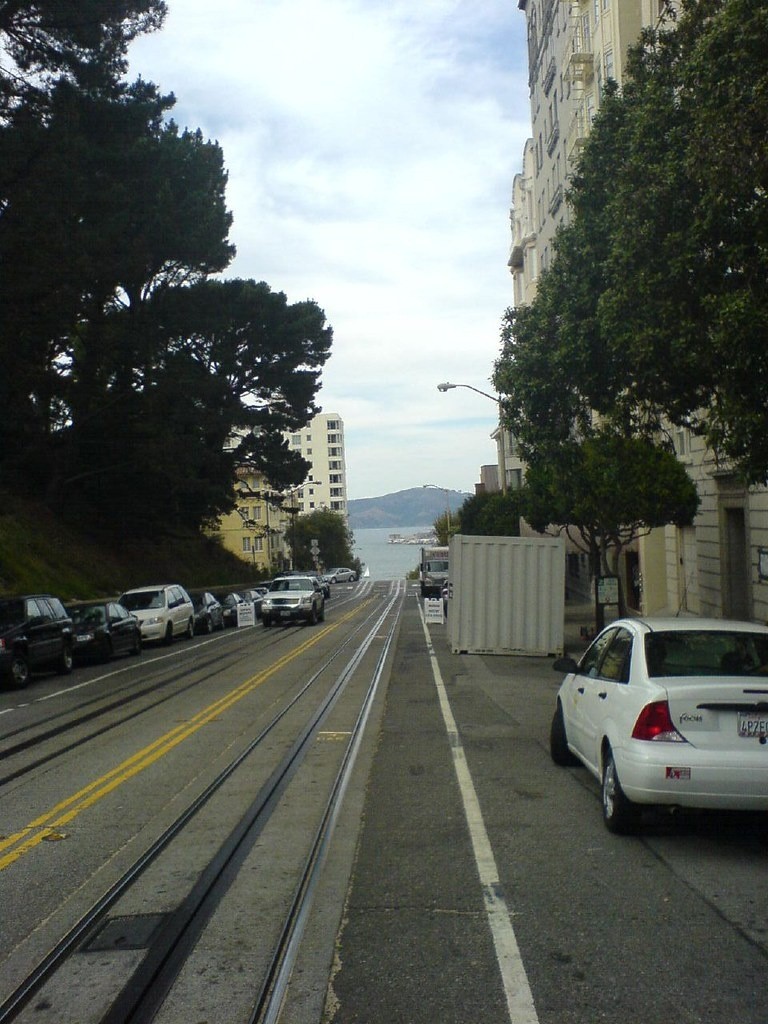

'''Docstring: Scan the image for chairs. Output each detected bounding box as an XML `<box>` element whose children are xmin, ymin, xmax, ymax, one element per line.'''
<box><xmin>721</xmin><ymin>649</ymin><xmax>758</xmax><ymax>676</ymax></box>
<box><xmin>616</xmin><ymin>645</ymin><xmax>669</xmax><ymax>684</ymax></box>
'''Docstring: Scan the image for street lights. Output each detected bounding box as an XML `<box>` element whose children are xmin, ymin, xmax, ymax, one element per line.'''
<box><xmin>423</xmin><ymin>483</ymin><xmax>451</xmax><ymax>542</ymax></box>
<box><xmin>434</xmin><ymin>381</ymin><xmax>509</xmax><ymax>497</ymax></box>
<box><xmin>291</xmin><ymin>481</ymin><xmax>324</xmax><ymax>567</ymax></box>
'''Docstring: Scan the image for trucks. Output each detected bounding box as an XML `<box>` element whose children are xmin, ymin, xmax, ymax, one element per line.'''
<box><xmin>419</xmin><ymin>545</ymin><xmax>449</xmax><ymax>598</ymax></box>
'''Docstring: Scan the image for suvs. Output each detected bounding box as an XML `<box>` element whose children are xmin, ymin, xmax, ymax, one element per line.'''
<box><xmin>116</xmin><ymin>584</ymin><xmax>195</xmax><ymax>646</ymax></box>
<box><xmin>0</xmin><ymin>594</ymin><xmax>77</xmax><ymax>689</ymax></box>
<box><xmin>259</xmin><ymin>576</ymin><xmax>325</xmax><ymax>624</ymax></box>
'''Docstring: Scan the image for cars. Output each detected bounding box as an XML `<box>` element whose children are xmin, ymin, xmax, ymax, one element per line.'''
<box><xmin>186</xmin><ymin>591</ymin><xmax>227</xmax><ymax>634</ymax></box>
<box><xmin>322</xmin><ymin>568</ymin><xmax>358</xmax><ymax>584</ymax></box>
<box><xmin>277</xmin><ymin>569</ymin><xmax>331</xmax><ymax>598</ymax></box>
<box><xmin>235</xmin><ymin>581</ymin><xmax>272</xmax><ymax>618</ymax></box>
<box><xmin>215</xmin><ymin>592</ymin><xmax>246</xmax><ymax>626</ymax></box>
<box><xmin>550</xmin><ymin>616</ymin><xmax>768</xmax><ymax>835</ymax></box>
<box><xmin>67</xmin><ymin>602</ymin><xmax>143</xmax><ymax>668</ymax></box>
<box><xmin>439</xmin><ymin>577</ymin><xmax>448</xmax><ymax>609</ymax></box>
